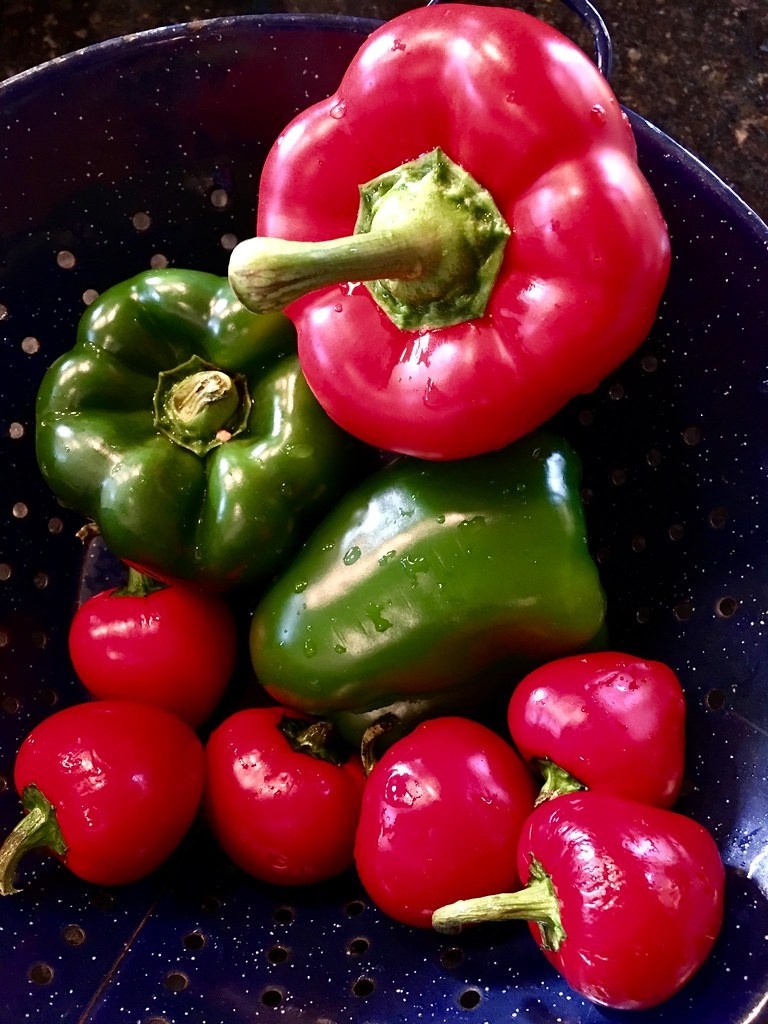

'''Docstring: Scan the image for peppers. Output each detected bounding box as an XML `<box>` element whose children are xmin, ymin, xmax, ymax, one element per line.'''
<box><xmin>1</xmin><ymin>7</ymin><xmax>727</xmax><ymax>1011</ymax></box>
